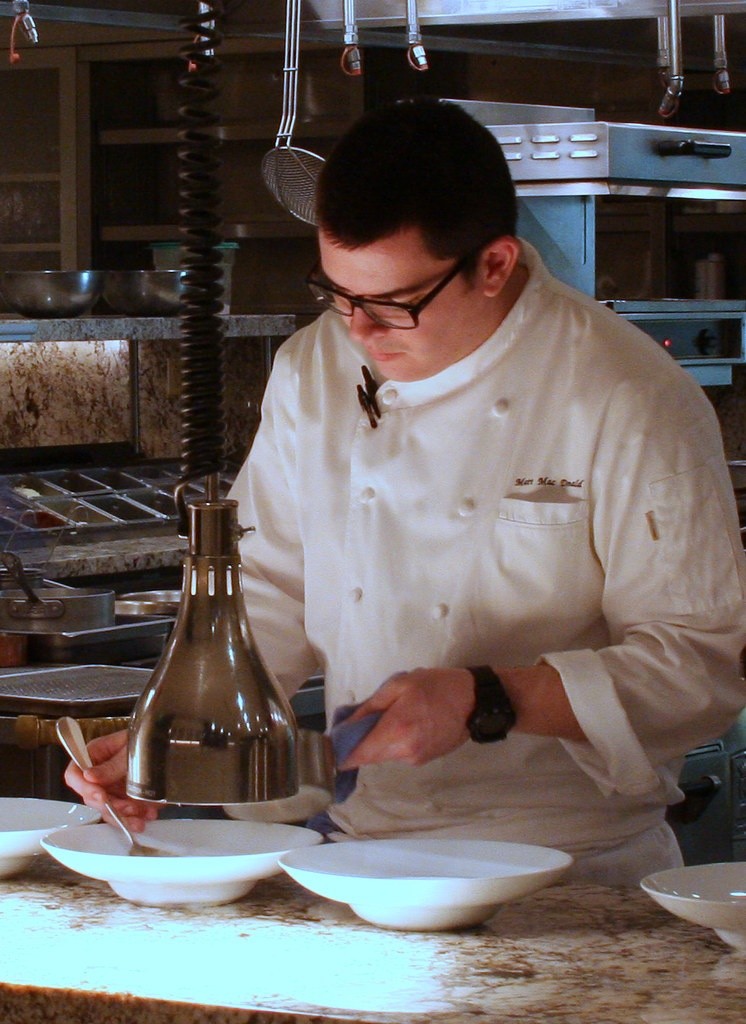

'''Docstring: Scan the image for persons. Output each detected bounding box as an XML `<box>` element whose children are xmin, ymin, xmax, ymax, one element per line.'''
<box><xmin>64</xmin><ymin>94</ymin><xmax>746</xmax><ymax>889</ymax></box>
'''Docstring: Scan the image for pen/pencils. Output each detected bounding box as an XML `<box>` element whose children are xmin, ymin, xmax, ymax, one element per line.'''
<box><xmin>357</xmin><ymin>384</ymin><xmax>377</xmax><ymax>429</ymax></box>
<box><xmin>361</xmin><ymin>365</ymin><xmax>382</xmax><ymax>419</ymax></box>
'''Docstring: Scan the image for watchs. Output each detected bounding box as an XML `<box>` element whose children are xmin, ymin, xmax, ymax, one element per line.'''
<box><xmin>465</xmin><ymin>665</ymin><xmax>516</xmax><ymax>744</ymax></box>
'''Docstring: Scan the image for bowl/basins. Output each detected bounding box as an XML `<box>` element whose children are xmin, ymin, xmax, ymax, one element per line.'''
<box><xmin>0</xmin><ymin>269</ymin><xmax>107</xmax><ymax>319</ymax></box>
<box><xmin>102</xmin><ymin>269</ymin><xmax>189</xmax><ymax>316</ymax></box>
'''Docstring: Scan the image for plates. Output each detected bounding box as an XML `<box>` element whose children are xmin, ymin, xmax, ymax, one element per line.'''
<box><xmin>40</xmin><ymin>818</ymin><xmax>322</xmax><ymax>908</ymax></box>
<box><xmin>0</xmin><ymin>797</ymin><xmax>102</xmax><ymax>879</ymax></box>
<box><xmin>277</xmin><ymin>838</ymin><xmax>575</xmax><ymax>931</ymax></box>
<box><xmin>641</xmin><ymin>859</ymin><xmax>746</xmax><ymax>948</ymax></box>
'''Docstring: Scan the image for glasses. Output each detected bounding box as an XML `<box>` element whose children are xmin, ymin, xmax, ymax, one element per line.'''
<box><xmin>304</xmin><ymin>254</ymin><xmax>467</xmax><ymax>330</ymax></box>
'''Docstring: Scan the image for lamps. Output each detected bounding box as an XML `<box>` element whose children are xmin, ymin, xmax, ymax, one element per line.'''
<box><xmin>125</xmin><ymin>0</ymin><xmax>305</xmax><ymax>806</ymax></box>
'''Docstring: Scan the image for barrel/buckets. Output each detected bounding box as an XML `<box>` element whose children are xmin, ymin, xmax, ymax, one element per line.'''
<box><xmin>0</xmin><ymin>586</ymin><xmax>117</xmax><ymax>632</ymax></box>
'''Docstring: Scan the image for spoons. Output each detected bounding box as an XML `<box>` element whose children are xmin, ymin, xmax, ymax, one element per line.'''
<box><xmin>55</xmin><ymin>716</ymin><xmax>177</xmax><ymax>857</ymax></box>
